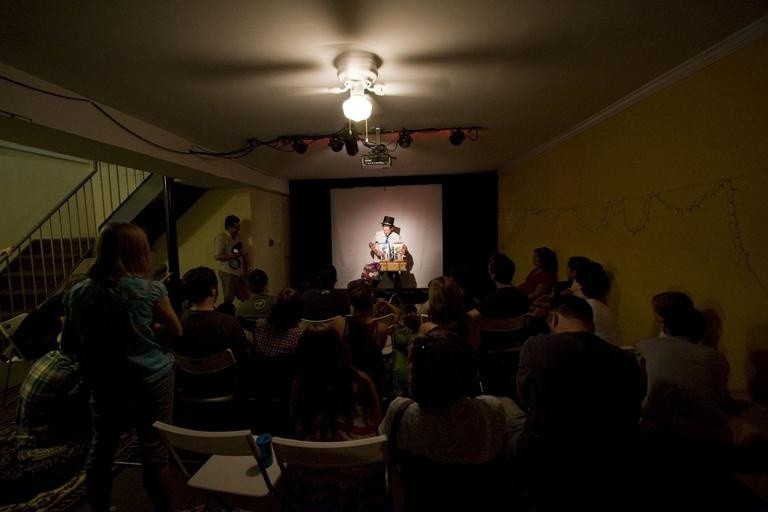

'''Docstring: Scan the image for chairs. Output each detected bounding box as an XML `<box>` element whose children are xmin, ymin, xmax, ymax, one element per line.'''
<box><xmin>270</xmin><ymin>433</ymin><xmax>390</xmax><ymax>512</ymax></box>
<box><xmin>154</xmin><ymin>423</ymin><xmax>287</xmax><ymax>511</ymax></box>
<box><xmin>0</xmin><ymin>309</ymin><xmax>526</xmax><ymax>411</ymax></box>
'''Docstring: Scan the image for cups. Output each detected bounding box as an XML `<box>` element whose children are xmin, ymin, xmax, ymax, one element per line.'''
<box><xmin>256</xmin><ymin>434</ymin><xmax>272</xmax><ymax>467</ymax></box>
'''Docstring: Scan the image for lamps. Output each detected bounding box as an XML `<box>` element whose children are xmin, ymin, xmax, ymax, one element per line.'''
<box><xmin>340</xmin><ymin>88</ymin><xmax>373</xmax><ymax>144</ymax></box>
<box><xmin>277</xmin><ymin>124</ymin><xmax>472</xmax><ymax>156</ymax></box>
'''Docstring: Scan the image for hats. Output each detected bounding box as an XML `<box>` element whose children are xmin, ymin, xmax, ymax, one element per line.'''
<box><xmin>381</xmin><ymin>216</ymin><xmax>395</xmax><ymax>227</ymax></box>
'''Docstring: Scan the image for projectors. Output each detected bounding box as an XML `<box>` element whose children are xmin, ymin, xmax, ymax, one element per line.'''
<box><xmin>361</xmin><ymin>153</ymin><xmax>394</xmax><ymax>170</ymax></box>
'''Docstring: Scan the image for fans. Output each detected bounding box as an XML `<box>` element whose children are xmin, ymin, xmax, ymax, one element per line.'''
<box><xmin>264</xmin><ymin>47</ymin><xmax>451</xmax><ymax>99</ymax></box>
